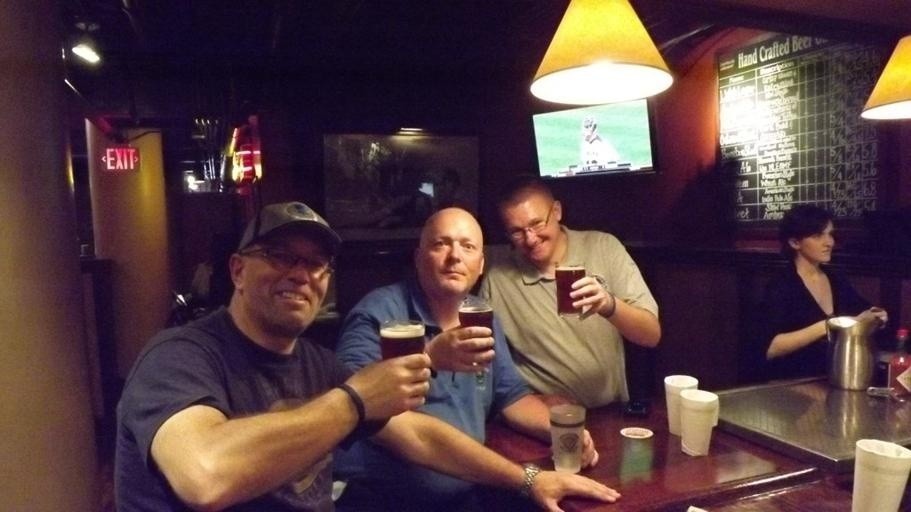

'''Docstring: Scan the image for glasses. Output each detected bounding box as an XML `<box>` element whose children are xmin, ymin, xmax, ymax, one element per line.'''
<box><xmin>242</xmin><ymin>248</ymin><xmax>334</xmax><ymax>276</ymax></box>
<box><xmin>507</xmin><ymin>200</ymin><xmax>555</xmax><ymax>241</ymax></box>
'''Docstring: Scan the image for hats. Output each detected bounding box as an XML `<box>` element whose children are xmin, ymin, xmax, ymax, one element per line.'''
<box><xmin>238</xmin><ymin>202</ymin><xmax>343</xmax><ymax>264</ymax></box>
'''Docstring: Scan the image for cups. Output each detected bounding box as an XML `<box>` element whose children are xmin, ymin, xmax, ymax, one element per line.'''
<box><xmin>680</xmin><ymin>390</ymin><xmax>718</xmax><ymax>456</ymax></box>
<box><xmin>378</xmin><ymin>318</ymin><xmax>425</xmax><ymax>365</ymax></box>
<box><xmin>852</xmin><ymin>439</ymin><xmax>911</xmax><ymax>512</ymax></box>
<box><xmin>555</xmin><ymin>262</ymin><xmax>585</xmax><ymax>317</ymax></box>
<box><xmin>665</xmin><ymin>376</ymin><xmax>698</xmax><ymax>436</ymax></box>
<box><xmin>459</xmin><ymin>298</ymin><xmax>495</xmax><ymax>363</ymax></box>
<box><xmin>551</xmin><ymin>402</ymin><xmax>586</xmax><ymax>474</ymax></box>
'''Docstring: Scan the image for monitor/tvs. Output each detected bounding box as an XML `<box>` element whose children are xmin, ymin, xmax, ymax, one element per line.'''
<box><xmin>532</xmin><ymin>96</ymin><xmax>657</xmax><ymax>189</ymax></box>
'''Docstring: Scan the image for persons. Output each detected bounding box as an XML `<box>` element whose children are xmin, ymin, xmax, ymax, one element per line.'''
<box><xmin>186</xmin><ymin>232</ymin><xmax>219</xmax><ymax>300</ymax></box>
<box><xmin>325</xmin><ymin>138</ymin><xmax>412</xmax><ymax>230</ymax></box>
<box><xmin>748</xmin><ymin>205</ymin><xmax>888</xmax><ymax>378</ymax></box>
<box><xmin>574</xmin><ymin>114</ymin><xmax>626</xmax><ymax>169</ymax></box>
<box><xmin>477</xmin><ymin>180</ymin><xmax>662</xmax><ymax>409</ymax></box>
<box><xmin>331</xmin><ymin>207</ymin><xmax>602</xmax><ymax>512</ymax></box>
<box><xmin>113</xmin><ymin>203</ymin><xmax>619</xmax><ymax>512</ymax></box>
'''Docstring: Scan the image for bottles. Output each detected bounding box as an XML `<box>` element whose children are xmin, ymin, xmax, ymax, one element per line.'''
<box><xmin>887</xmin><ymin>327</ymin><xmax>911</xmax><ymax>398</ymax></box>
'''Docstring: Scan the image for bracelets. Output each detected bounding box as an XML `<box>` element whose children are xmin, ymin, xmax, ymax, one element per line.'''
<box><xmin>520</xmin><ymin>462</ymin><xmax>540</xmax><ymax>500</ymax></box>
<box><xmin>422</xmin><ymin>353</ymin><xmax>438</xmax><ymax>381</ymax></box>
<box><xmin>599</xmin><ymin>292</ymin><xmax>615</xmax><ymax>319</ymax></box>
<box><xmin>337</xmin><ymin>383</ymin><xmax>365</xmax><ymax>427</ymax></box>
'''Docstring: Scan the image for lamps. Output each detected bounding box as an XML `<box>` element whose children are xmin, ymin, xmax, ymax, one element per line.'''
<box><xmin>858</xmin><ymin>36</ymin><xmax>911</xmax><ymax>120</ymax></box>
<box><xmin>529</xmin><ymin>0</ymin><xmax>675</xmax><ymax>106</ymax></box>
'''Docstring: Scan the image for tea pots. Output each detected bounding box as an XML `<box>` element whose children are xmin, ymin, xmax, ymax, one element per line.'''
<box><xmin>826</xmin><ymin>314</ymin><xmax>882</xmax><ymax>391</ymax></box>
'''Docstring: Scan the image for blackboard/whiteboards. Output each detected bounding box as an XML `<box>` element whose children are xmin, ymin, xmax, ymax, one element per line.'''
<box><xmin>714</xmin><ymin>32</ymin><xmax>899</xmax><ymax>227</ymax></box>
